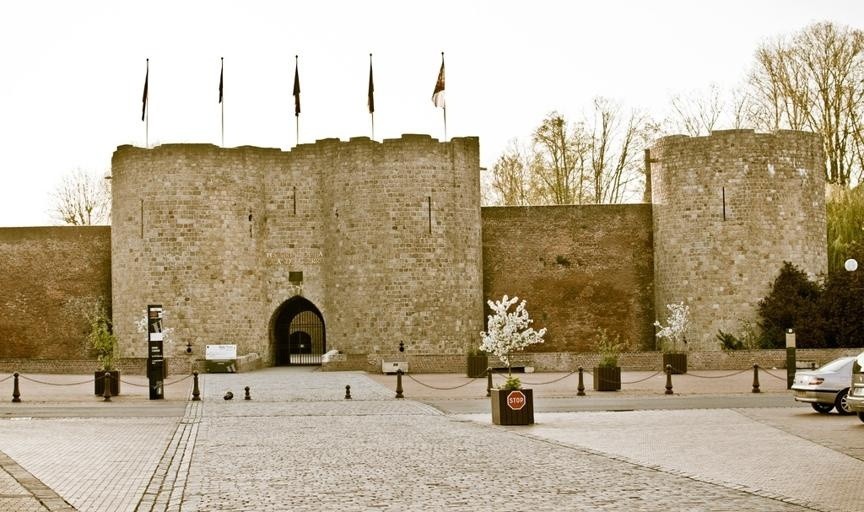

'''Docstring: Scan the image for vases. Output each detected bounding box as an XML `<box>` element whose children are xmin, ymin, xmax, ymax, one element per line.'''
<box><xmin>490</xmin><ymin>388</ymin><xmax>534</xmax><ymax>425</ymax></box>
<box><xmin>663</xmin><ymin>354</ymin><xmax>687</xmax><ymax>374</ymax></box>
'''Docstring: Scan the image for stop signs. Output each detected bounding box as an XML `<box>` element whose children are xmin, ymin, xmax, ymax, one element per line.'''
<box><xmin>507</xmin><ymin>391</ymin><xmax>527</xmax><ymax>411</ymax></box>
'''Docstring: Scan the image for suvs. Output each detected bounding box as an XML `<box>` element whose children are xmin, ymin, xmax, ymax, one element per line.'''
<box><xmin>847</xmin><ymin>353</ymin><xmax>864</xmax><ymax>422</ymax></box>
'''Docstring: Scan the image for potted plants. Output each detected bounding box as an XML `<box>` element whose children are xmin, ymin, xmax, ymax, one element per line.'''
<box><xmin>87</xmin><ymin>311</ymin><xmax>120</xmax><ymax>395</ymax></box>
<box><xmin>593</xmin><ymin>329</ymin><xmax>627</xmax><ymax>390</ymax></box>
<box><xmin>464</xmin><ymin>333</ymin><xmax>489</xmax><ymax>379</ymax></box>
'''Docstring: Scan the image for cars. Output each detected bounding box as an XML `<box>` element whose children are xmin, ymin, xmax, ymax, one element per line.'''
<box><xmin>792</xmin><ymin>356</ymin><xmax>859</xmax><ymax>414</ymax></box>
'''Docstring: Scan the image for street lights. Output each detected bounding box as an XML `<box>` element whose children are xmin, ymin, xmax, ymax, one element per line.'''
<box><xmin>842</xmin><ymin>259</ymin><xmax>860</xmax><ymax>284</ymax></box>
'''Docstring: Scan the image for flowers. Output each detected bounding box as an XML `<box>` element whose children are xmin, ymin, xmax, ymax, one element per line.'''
<box><xmin>479</xmin><ymin>295</ymin><xmax>547</xmax><ymax>388</ymax></box>
<box><xmin>653</xmin><ymin>301</ymin><xmax>692</xmax><ymax>354</ymax></box>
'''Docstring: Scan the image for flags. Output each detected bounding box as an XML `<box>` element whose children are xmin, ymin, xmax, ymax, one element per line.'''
<box><xmin>219</xmin><ymin>57</ymin><xmax>224</xmax><ymax>103</ymax></box>
<box><xmin>368</xmin><ymin>53</ymin><xmax>374</xmax><ymax>113</ymax></box>
<box><xmin>293</xmin><ymin>55</ymin><xmax>300</xmax><ymax>116</ymax></box>
<box><xmin>142</xmin><ymin>58</ymin><xmax>149</xmax><ymax>121</ymax></box>
<box><xmin>431</xmin><ymin>52</ymin><xmax>446</xmax><ymax>108</ymax></box>
